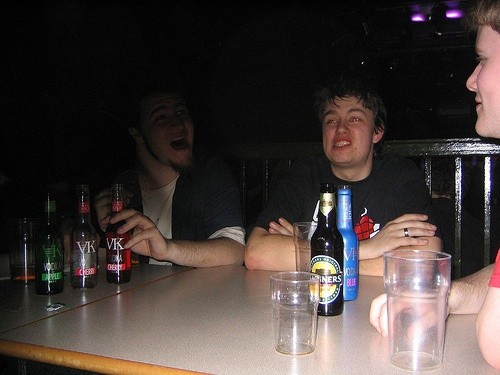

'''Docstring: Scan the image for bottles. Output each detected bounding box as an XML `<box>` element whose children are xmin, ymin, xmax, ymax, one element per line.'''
<box><xmin>307</xmin><ymin>183</ymin><xmax>344</xmax><ymax>317</ymax></box>
<box><xmin>70</xmin><ymin>184</ymin><xmax>98</xmax><ymax>289</ymax></box>
<box><xmin>332</xmin><ymin>184</ymin><xmax>359</xmax><ymax>300</ymax></box>
<box><xmin>34</xmin><ymin>189</ymin><xmax>65</xmax><ymax>295</ymax></box>
<box><xmin>104</xmin><ymin>182</ymin><xmax>132</xmax><ymax>284</ymax></box>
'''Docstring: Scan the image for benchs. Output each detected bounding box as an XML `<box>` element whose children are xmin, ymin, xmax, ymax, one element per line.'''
<box><xmin>199</xmin><ymin>136</ymin><xmax>500</xmax><ymax>280</ymax></box>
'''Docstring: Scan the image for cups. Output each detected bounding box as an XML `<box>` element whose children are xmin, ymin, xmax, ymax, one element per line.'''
<box><xmin>8</xmin><ymin>217</ymin><xmax>38</xmax><ymax>281</ymax></box>
<box><xmin>269</xmin><ymin>270</ymin><xmax>320</xmax><ymax>355</ymax></box>
<box><xmin>384</xmin><ymin>249</ymin><xmax>453</xmax><ymax>371</ymax></box>
<box><xmin>293</xmin><ymin>222</ymin><xmax>321</xmax><ymax>298</ymax></box>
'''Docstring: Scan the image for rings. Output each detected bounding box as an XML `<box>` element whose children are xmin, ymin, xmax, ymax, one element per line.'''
<box><xmin>404</xmin><ymin>228</ymin><xmax>409</xmax><ymax>237</ymax></box>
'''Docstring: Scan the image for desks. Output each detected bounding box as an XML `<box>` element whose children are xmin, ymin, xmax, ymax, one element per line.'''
<box><xmin>0</xmin><ymin>265</ymin><xmax>498</xmax><ymax>375</ymax></box>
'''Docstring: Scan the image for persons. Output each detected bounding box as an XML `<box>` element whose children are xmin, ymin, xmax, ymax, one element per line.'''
<box><xmin>244</xmin><ymin>72</ymin><xmax>442</xmax><ymax>277</ymax></box>
<box><xmin>93</xmin><ymin>80</ymin><xmax>246</xmax><ymax>267</ymax></box>
<box><xmin>368</xmin><ymin>0</ymin><xmax>500</xmax><ymax>370</ymax></box>
